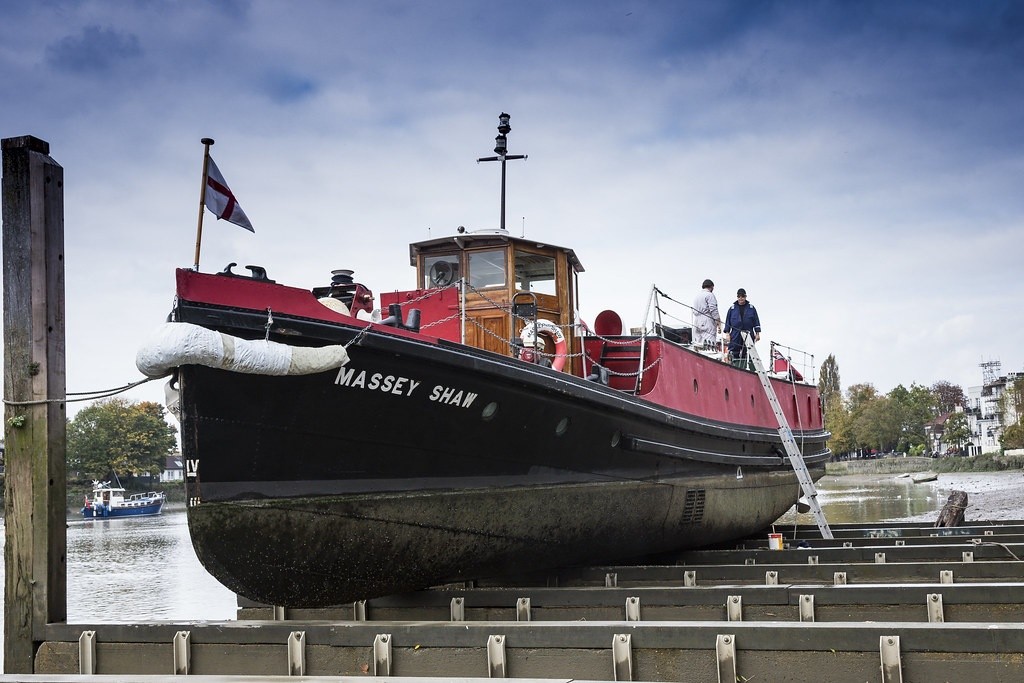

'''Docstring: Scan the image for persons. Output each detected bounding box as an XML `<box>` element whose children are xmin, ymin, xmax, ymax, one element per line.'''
<box><xmin>723</xmin><ymin>288</ymin><xmax>761</xmax><ymax>370</ymax></box>
<box><xmin>921</xmin><ymin>449</ymin><xmax>938</xmax><ymax>457</ymax></box>
<box><xmin>692</xmin><ymin>279</ymin><xmax>721</xmax><ymax>349</ymax></box>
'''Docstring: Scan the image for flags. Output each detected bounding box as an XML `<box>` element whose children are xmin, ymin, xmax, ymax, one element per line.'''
<box><xmin>774</xmin><ymin>351</ymin><xmax>804</xmax><ymax>381</ymax></box>
<box><xmin>203</xmin><ymin>155</ymin><xmax>255</xmax><ymax>232</ymax></box>
<box><xmin>85</xmin><ymin>497</ymin><xmax>91</xmax><ymax>510</ymax></box>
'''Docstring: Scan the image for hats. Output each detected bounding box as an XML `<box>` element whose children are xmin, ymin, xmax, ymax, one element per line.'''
<box><xmin>737</xmin><ymin>289</ymin><xmax>746</xmax><ymax>294</ymax></box>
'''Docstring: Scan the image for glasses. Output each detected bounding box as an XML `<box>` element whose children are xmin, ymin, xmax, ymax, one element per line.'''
<box><xmin>737</xmin><ymin>295</ymin><xmax>747</xmax><ymax>297</ymax></box>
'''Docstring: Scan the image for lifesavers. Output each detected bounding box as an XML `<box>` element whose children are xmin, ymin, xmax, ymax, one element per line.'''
<box><xmin>515</xmin><ymin>318</ymin><xmax>567</xmax><ymax>372</ymax></box>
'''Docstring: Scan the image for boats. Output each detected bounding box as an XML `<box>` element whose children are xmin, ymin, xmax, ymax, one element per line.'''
<box><xmin>79</xmin><ymin>479</ymin><xmax>166</xmax><ymax>517</ymax></box>
<box><xmin>133</xmin><ymin>112</ymin><xmax>835</xmax><ymax>605</ymax></box>
